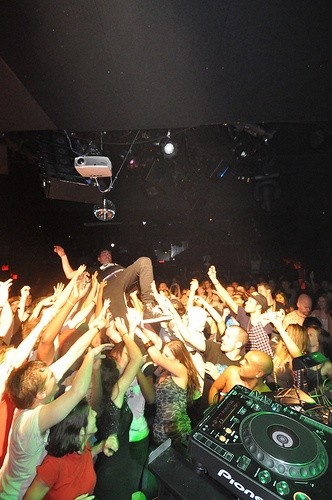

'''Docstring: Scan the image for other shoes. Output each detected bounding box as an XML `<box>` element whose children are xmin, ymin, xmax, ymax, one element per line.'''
<box><xmin>142</xmin><ymin>307</ymin><xmax>173</xmax><ymax>323</ymax></box>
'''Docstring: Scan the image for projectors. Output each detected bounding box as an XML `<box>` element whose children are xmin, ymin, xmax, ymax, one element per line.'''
<box><xmin>74</xmin><ymin>156</ymin><xmax>112</xmax><ymax>179</ymax></box>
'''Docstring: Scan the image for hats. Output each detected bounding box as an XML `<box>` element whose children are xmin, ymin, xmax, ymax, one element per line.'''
<box><xmin>245</xmin><ymin>290</ymin><xmax>269</xmax><ymax>313</ymax></box>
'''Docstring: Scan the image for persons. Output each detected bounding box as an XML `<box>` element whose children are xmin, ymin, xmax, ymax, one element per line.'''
<box><xmin>0</xmin><ymin>264</ymin><xmax>332</xmax><ymax>500</ymax></box>
<box><xmin>54</xmin><ymin>246</ymin><xmax>174</xmax><ymax>335</ymax></box>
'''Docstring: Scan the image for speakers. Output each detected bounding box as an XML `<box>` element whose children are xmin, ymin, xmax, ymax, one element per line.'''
<box><xmin>39</xmin><ymin>163</ymin><xmax>105</xmax><ymax>205</ymax></box>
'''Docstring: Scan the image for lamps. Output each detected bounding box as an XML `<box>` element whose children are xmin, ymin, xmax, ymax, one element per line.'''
<box><xmin>156</xmin><ymin>132</ymin><xmax>175</xmax><ymax>155</ymax></box>
